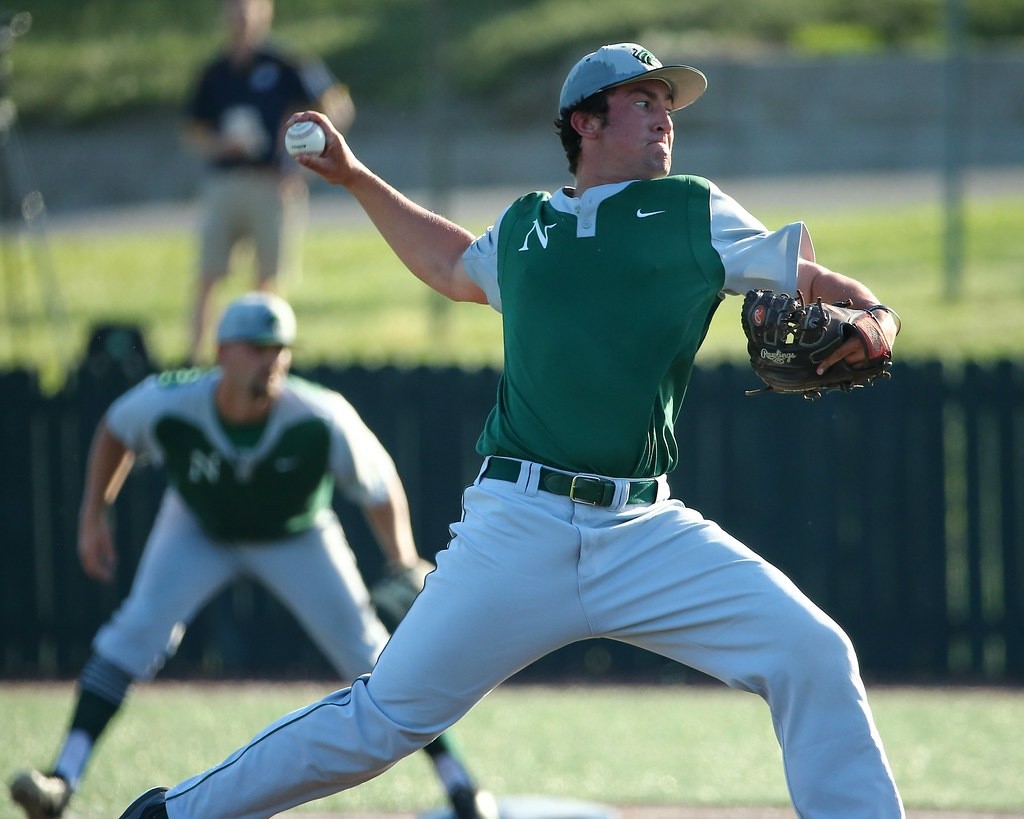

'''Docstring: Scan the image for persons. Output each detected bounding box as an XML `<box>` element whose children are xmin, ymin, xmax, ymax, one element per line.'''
<box><xmin>119</xmin><ymin>42</ymin><xmax>902</xmax><ymax>819</ymax></box>
<box><xmin>9</xmin><ymin>291</ymin><xmax>474</xmax><ymax>819</ymax></box>
<box><xmin>183</xmin><ymin>0</ymin><xmax>355</xmax><ymax>370</ymax></box>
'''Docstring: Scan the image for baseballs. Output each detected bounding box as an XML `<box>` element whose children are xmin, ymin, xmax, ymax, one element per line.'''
<box><xmin>284</xmin><ymin>120</ymin><xmax>326</xmax><ymax>159</ymax></box>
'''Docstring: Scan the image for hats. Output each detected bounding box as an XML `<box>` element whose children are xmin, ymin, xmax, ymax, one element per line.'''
<box><xmin>559</xmin><ymin>42</ymin><xmax>708</xmax><ymax>113</ymax></box>
<box><xmin>219</xmin><ymin>294</ymin><xmax>299</xmax><ymax>348</ymax></box>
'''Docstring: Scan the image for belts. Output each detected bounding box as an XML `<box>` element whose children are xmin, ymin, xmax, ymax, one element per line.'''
<box><xmin>483</xmin><ymin>451</ymin><xmax>658</xmax><ymax>508</ymax></box>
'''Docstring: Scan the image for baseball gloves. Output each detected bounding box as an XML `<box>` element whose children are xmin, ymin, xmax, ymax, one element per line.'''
<box><xmin>742</xmin><ymin>289</ymin><xmax>893</xmax><ymax>396</ymax></box>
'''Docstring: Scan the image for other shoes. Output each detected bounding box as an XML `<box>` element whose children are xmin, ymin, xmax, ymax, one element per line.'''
<box><xmin>118</xmin><ymin>784</ymin><xmax>172</xmax><ymax>819</ymax></box>
<box><xmin>449</xmin><ymin>779</ymin><xmax>499</xmax><ymax>819</ymax></box>
<box><xmin>15</xmin><ymin>770</ymin><xmax>69</xmax><ymax>819</ymax></box>
